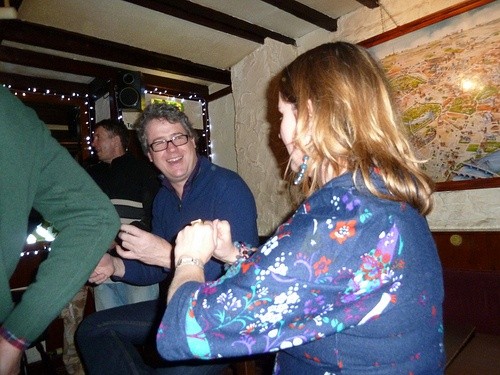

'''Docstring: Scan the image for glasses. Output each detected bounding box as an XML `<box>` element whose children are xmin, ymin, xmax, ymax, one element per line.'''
<box><xmin>149</xmin><ymin>134</ymin><xmax>190</xmax><ymax>152</ymax></box>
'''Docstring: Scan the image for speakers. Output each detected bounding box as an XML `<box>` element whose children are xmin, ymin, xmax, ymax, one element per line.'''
<box><xmin>117</xmin><ymin>71</ymin><xmax>142</xmax><ymax>112</ymax></box>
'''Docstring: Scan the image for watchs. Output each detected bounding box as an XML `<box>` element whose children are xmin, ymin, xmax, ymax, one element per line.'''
<box><xmin>174</xmin><ymin>257</ymin><xmax>205</xmax><ymax>269</ymax></box>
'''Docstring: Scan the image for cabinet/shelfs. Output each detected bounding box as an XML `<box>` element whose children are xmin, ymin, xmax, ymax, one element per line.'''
<box><xmin>428</xmin><ymin>229</ymin><xmax>500</xmax><ymax>334</ymax></box>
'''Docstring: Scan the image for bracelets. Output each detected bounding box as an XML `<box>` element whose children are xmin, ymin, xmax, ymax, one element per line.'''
<box><xmin>224</xmin><ymin>241</ymin><xmax>252</xmax><ymax>273</ymax></box>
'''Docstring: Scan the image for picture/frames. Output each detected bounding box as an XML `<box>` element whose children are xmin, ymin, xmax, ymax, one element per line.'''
<box><xmin>355</xmin><ymin>0</ymin><xmax>500</xmax><ymax>192</ymax></box>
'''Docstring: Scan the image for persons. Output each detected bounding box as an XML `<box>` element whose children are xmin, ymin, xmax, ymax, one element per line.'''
<box><xmin>58</xmin><ymin>285</ymin><xmax>94</xmax><ymax>375</ymax></box>
<box><xmin>82</xmin><ymin>118</ymin><xmax>163</xmax><ymax>314</ymax></box>
<box><xmin>155</xmin><ymin>41</ymin><xmax>447</xmax><ymax>375</ymax></box>
<box><xmin>72</xmin><ymin>100</ymin><xmax>259</xmax><ymax>375</ymax></box>
<box><xmin>1</xmin><ymin>82</ymin><xmax>122</xmax><ymax>375</ymax></box>
